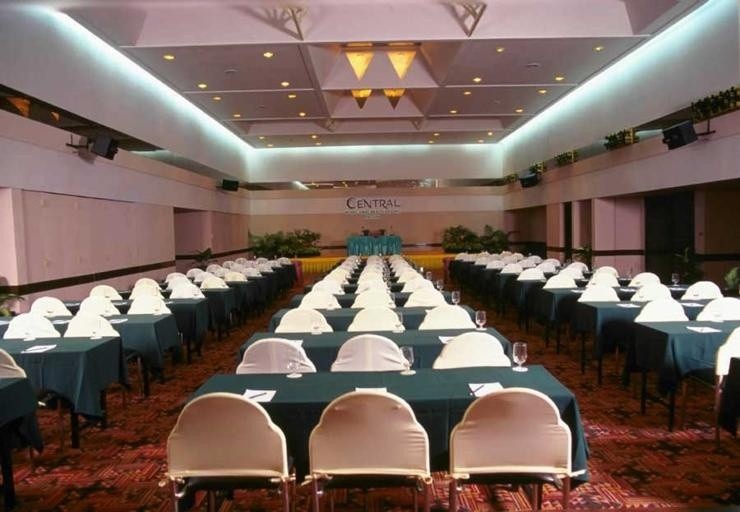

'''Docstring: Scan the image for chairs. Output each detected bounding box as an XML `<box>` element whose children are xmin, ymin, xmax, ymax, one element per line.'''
<box><xmin>226</xmin><ymin>272</ymin><xmax>246</xmax><ymax>282</ymax></box>
<box><xmin>536</xmin><ymin>262</ymin><xmax>556</xmax><ymax>273</ymax></box>
<box><xmin>587</xmin><ymin>270</ymin><xmax>619</xmax><ymax>285</ymax></box>
<box><xmin>87</xmin><ymin>283</ymin><xmax>116</xmax><ymax>296</ymax></box>
<box><xmin>544</xmin><ymin>259</ymin><xmax>560</xmax><ymax>267</ymax></box>
<box><xmin>577</xmin><ymin>283</ymin><xmax>616</xmax><ymax>303</ymax></box>
<box><xmin>628</xmin><ymin>272</ymin><xmax>660</xmax><ymax>286</ymax></box>
<box><xmin>357</xmin><ymin>271</ymin><xmax>383</xmax><ymax>284</ymax></box>
<box><xmin>433</xmin><ymin>332</ymin><xmax>512</xmax><ymax>370</ymax></box>
<box><xmin>244</xmin><ymin>268</ymin><xmax>261</xmax><ymax>277</ymax></box>
<box><xmin>501</xmin><ymin>263</ymin><xmax>522</xmax><ymax>273</ymax></box>
<box><xmin>308</xmin><ymin>392</ymin><xmax>431</xmax><ymax>511</ymax></box>
<box><xmin>331</xmin><ymin>334</ymin><xmax>410</xmax><ymax>372</ymax></box>
<box><xmin>26</xmin><ymin>294</ymin><xmax>63</xmax><ymax>315</ymax></box>
<box><xmin>237</xmin><ymin>258</ymin><xmax>248</xmax><ymax>266</ymax></box>
<box><xmin>517</xmin><ymin>268</ymin><xmax>540</xmax><ymax>280</ymax></box>
<box><xmin>567</xmin><ymin>262</ymin><xmax>588</xmax><ymax>272</ymax></box>
<box><xmin>61</xmin><ymin>306</ymin><xmax>115</xmax><ymax>338</ymax></box>
<box><xmin>353</xmin><ymin>289</ymin><xmax>395</xmax><ymax>308</ymax></box>
<box><xmin>277</xmin><ymin>257</ymin><xmax>292</xmax><ymax>265</ymax></box>
<box><xmin>397</xmin><ymin>271</ymin><xmax>423</xmax><ymax>284</ymax></box>
<box><xmin>258</xmin><ymin>263</ymin><xmax>273</xmax><ymax>273</ymax></box>
<box><xmin>348</xmin><ymin>304</ymin><xmax>406</xmax><ymax>333</ymax></box>
<box><xmin>716</xmin><ymin>331</ymin><xmax>731</xmax><ymax>373</ymax></box>
<box><xmin>256</xmin><ymin>258</ymin><xmax>268</xmax><ymax>264</ymax></box>
<box><xmin>267</xmin><ymin>261</ymin><xmax>282</xmax><ymax>268</ymax></box>
<box><xmin>128</xmin><ymin>291</ymin><xmax>164</xmax><ymax>319</ymax></box>
<box><xmin>273</xmin><ymin>308</ymin><xmax>333</xmax><ymax>334</ymax></box>
<box><xmin>404</xmin><ymin>287</ymin><xmax>445</xmax><ymax>306</ymax></box>
<box><xmin>129</xmin><ymin>282</ymin><xmax>160</xmax><ymax>298</ymax></box>
<box><xmin>516</xmin><ymin>259</ymin><xmax>536</xmax><ymax>267</ymax></box>
<box><xmin>169</xmin><ymin>282</ymin><xmax>201</xmax><ymax>298</ymax></box>
<box><xmin>634</xmin><ymin>297</ymin><xmax>687</xmax><ymax>323</ymax></box>
<box><xmin>164</xmin><ymin>392</ymin><xmax>290</xmax><ymax>512</ymax></box>
<box><xmin>686</xmin><ymin>278</ymin><xmax>722</xmax><ymax>300</ymax></box>
<box><xmin>632</xmin><ymin>283</ymin><xmax>669</xmax><ymax>303</ymax></box>
<box><xmin>301</xmin><ymin>293</ymin><xmax>340</xmax><ymax>310</ymax></box>
<box><xmin>312</xmin><ymin>280</ymin><xmax>344</xmax><ymax>294</ymax></box>
<box><xmin>543</xmin><ymin>273</ymin><xmax>573</xmax><ymax>289</ymax></box>
<box><xmin>207</xmin><ymin>265</ymin><xmax>223</xmax><ymax>273</ymax></box>
<box><xmin>236</xmin><ymin>337</ymin><xmax>316</xmax><ymax>375</ymax></box>
<box><xmin>487</xmin><ymin>260</ymin><xmax>503</xmax><ymax>268</ymax></box>
<box><xmin>475</xmin><ymin>257</ymin><xmax>491</xmax><ymax>265</ymax></box>
<box><xmin>324</xmin><ymin>274</ymin><xmax>348</xmax><ymax>284</ymax></box>
<box><xmin>223</xmin><ymin>261</ymin><xmax>235</xmax><ymax>270</ymax></box>
<box><xmin>560</xmin><ymin>267</ymin><xmax>582</xmax><ymax>280</ymax></box>
<box><xmin>165</xmin><ymin>276</ymin><xmax>191</xmax><ymax>288</ymax></box>
<box><xmin>132</xmin><ymin>276</ymin><xmax>162</xmax><ymax>289</ymax></box>
<box><xmin>187</xmin><ymin>268</ymin><xmax>205</xmax><ymax>278</ymax></box>
<box><xmin>4</xmin><ymin>313</ymin><xmax>58</xmax><ymax>339</ymax></box>
<box><xmin>199</xmin><ymin>275</ymin><xmax>228</xmax><ymax>288</ymax></box>
<box><xmin>418</xmin><ymin>302</ymin><xmax>473</xmax><ymax>329</ymax></box>
<box><xmin>446</xmin><ymin>388</ymin><xmax>574</xmax><ymax>511</ymax></box>
<box><xmin>528</xmin><ymin>255</ymin><xmax>542</xmax><ymax>263</ymax></box>
<box><xmin>0</xmin><ymin>341</ymin><xmax>23</xmax><ymax>383</ymax></box>
<box><xmin>80</xmin><ymin>294</ymin><xmax>114</xmax><ymax>317</ymax></box>
<box><xmin>594</xmin><ymin>266</ymin><xmax>616</xmax><ymax>275</ymax></box>
<box><xmin>194</xmin><ymin>272</ymin><xmax>214</xmax><ymax>282</ymax></box>
<box><xmin>401</xmin><ymin>278</ymin><xmax>434</xmax><ymax>292</ymax></box>
<box><xmin>355</xmin><ymin>278</ymin><xmax>390</xmax><ymax>292</ymax></box>
<box><xmin>695</xmin><ymin>297</ymin><xmax>732</xmax><ymax>320</ymax></box>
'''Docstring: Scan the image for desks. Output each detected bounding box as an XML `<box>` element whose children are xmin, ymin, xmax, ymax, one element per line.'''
<box><xmin>62</xmin><ymin>297</ymin><xmax>210</xmax><ymax>358</ymax></box>
<box><xmin>119</xmin><ymin>279</ymin><xmax>256</xmax><ymax>328</ymax></box>
<box><xmin>79</xmin><ymin>288</ymin><xmax>237</xmax><ymax>340</ymax></box>
<box><xmin>240</xmin><ymin>326</ymin><xmax>511</xmax><ymax>368</ymax></box>
<box><xmin>182</xmin><ymin>365</ymin><xmax>590</xmax><ymax>512</ymax></box>
<box><xmin>497</xmin><ymin>273</ymin><xmax>519</xmax><ymax>318</ymax></box>
<box><xmin>480</xmin><ymin>268</ymin><xmax>502</xmax><ymax>310</ymax></box>
<box><xmin>536</xmin><ymin>286</ymin><xmax>593</xmax><ymax>350</ymax></box>
<box><xmin>0</xmin><ymin>310</ymin><xmax>177</xmax><ymax>395</ymax></box>
<box><xmin>470</xmin><ymin>263</ymin><xmax>486</xmax><ymax>303</ymax></box>
<box><xmin>573</xmin><ymin>295</ymin><xmax>638</xmax><ymax>386</ymax></box>
<box><xmin>621</xmin><ymin>320</ymin><xmax>676</xmax><ymax>415</ymax></box>
<box><xmin>291</xmin><ymin>286</ymin><xmax>453</xmax><ymax>307</ymax></box>
<box><xmin>516</xmin><ymin>276</ymin><xmax>559</xmax><ymax>333</ymax></box>
<box><xmin>1</xmin><ymin>334</ymin><xmax>119</xmax><ymax>445</ymax></box>
<box><xmin>0</xmin><ymin>376</ymin><xmax>44</xmax><ymax>512</ymax></box>
<box><xmin>272</xmin><ymin>302</ymin><xmax>478</xmax><ymax>332</ymax></box>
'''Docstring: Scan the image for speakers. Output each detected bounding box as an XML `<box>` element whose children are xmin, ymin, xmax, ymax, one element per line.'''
<box><xmin>223</xmin><ymin>180</ymin><xmax>238</xmax><ymax>191</ymax></box>
<box><xmin>91</xmin><ymin>135</ymin><xmax>119</xmax><ymax>160</ymax></box>
<box><xmin>663</xmin><ymin>120</ymin><xmax>697</xmax><ymax>150</ymax></box>
<box><xmin>521</xmin><ymin>173</ymin><xmax>537</xmax><ymax>187</ymax></box>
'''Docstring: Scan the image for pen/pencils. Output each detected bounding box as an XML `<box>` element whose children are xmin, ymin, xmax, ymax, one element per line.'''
<box><xmin>248</xmin><ymin>392</ymin><xmax>267</xmax><ymax>399</ymax></box>
<box><xmin>469</xmin><ymin>384</ymin><xmax>484</xmax><ymax>395</ymax></box>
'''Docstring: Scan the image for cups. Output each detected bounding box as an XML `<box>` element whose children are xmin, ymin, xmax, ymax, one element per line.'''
<box><xmin>385</xmin><ymin>280</ymin><xmax>392</xmax><ymax>293</ymax></box>
<box><xmin>191</xmin><ymin>286</ymin><xmax>200</xmax><ymax>299</ymax></box>
<box><xmin>386</xmin><ymin>293</ymin><xmax>396</xmax><ymax>310</ymax></box>
<box><xmin>88</xmin><ymin>315</ymin><xmax>102</xmax><ymax>339</ymax></box>
<box><xmin>398</xmin><ymin>346</ymin><xmax>416</xmax><ymax>376</ymax></box>
<box><xmin>311</xmin><ymin>316</ymin><xmax>323</xmax><ymax>336</ymax></box>
<box><xmin>393</xmin><ymin>312</ymin><xmax>403</xmax><ymax>333</ymax></box>
<box><xmin>451</xmin><ymin>291</ymin><xmax>460</xmax><ymax>306</ymax></box>
<box><xmin>624</xmin><ymin>266</ymin><xmax>632</xmax><ymax>281</ymax></box>
<box><xmin>285</xmin><ymin>347</ymin><xmax>302</xmax><ymax>379</ymax></box>
<box><xmin>512</xmin><ymin>343</ymin><xmax>529</xmax><ymax>373</ymax></box>
<box><xmin>437</xmin><ymin>280</ymin><xmax>444</xmax><ymax>292</ymax></box>
<box><xmin>475</xmin><ymin>311</ymin><xmax>487</xmax><ymax>330</ymax></box>
<box><xmin>152</xmin><ymin>299</ymin><xmax>161</xmax><ymax>316</ymax></box>
<box><xmin>672</xmin><ymin>273</ymin><xmax>680</xmax><ymax>287</ymax></box>
<box><xmin>426</xmin><ymin>272</ymin><xmax>432</xmax><ymax>280</ymax></box>
